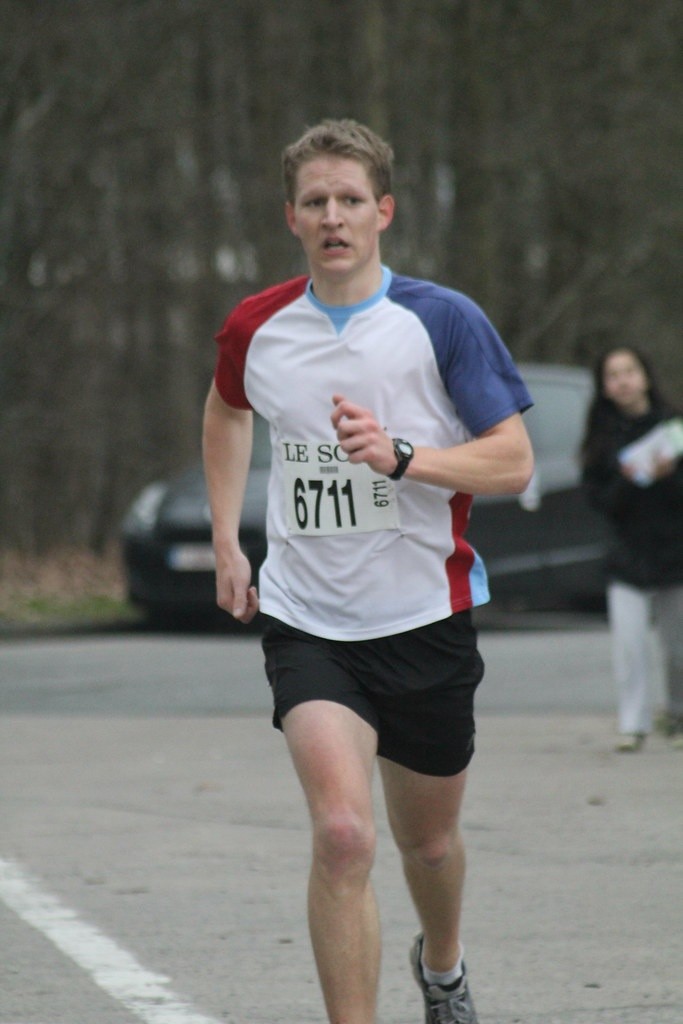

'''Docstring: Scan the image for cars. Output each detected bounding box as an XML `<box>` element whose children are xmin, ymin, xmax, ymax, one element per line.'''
<box><xmin>119</xmin><ymin>361</ymin><xmax>609</xmax><ymax>635</ymax></box>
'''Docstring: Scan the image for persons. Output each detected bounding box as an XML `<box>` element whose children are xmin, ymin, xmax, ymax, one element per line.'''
<box><xmin>577</xmin><ymin>343</ymin><xmax>683</xmax><ymax>751</ymax></box>
<box><xmin>204</xmin><ymin>120</ymin><xmax>535</xmax><ymax>1024</ymax></box>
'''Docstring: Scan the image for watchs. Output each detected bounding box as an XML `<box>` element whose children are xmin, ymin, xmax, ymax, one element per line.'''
<box><xmin>387</xmin><ymin>438</ymin><xmax>414</xmax><ymax>481</ymax></box>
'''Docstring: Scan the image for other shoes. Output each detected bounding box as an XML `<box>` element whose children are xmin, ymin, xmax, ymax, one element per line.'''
<box><xmin>617</xmin><ymin>732</ymin><xmax>645</xmax><ymax>752</ymax></box>
<box><xmin>652</xmin><ymin>713</ymin><xmax>679</xmax><ymax>736</ymax></box>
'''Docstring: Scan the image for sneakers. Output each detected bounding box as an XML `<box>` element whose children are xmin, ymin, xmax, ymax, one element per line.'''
<box><xmin>409</xmin><ymin>932</ymin><xmax>479</xmax><ymax>1024</ymax></box>
